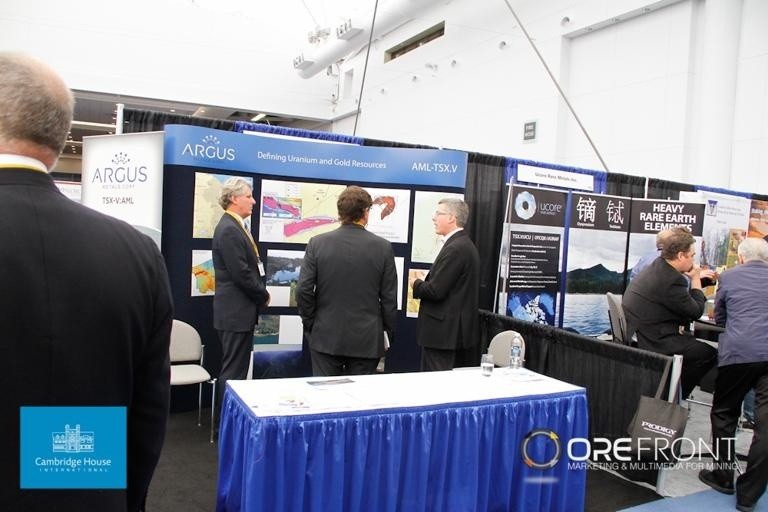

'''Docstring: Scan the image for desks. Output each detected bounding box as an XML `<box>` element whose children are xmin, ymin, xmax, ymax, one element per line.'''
<box><xmin>678</xmin><ymin>314</ymin><xmax>746</xmax><ymax>433</ymax></box>
<box><xmin>215</xmin><ymin>362</ymin><xmax>592</xmax><ymax>511</ymax></box>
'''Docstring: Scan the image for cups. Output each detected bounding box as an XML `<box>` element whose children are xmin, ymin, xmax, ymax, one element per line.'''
<box><xmin>481</xmin><ymin>353</ymin><xmax>494</xmax><ymax>377</ymax></box>
<box><xmin>706</xmin><ymin>300</ymin><xmax>717</xmax><ymax>321</ymax></box>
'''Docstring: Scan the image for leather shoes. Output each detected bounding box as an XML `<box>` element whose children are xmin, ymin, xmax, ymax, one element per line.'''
<box><xmin>698</xmin><ymin>468</ymin><xmax>734</xmax><ymax>494</ymax></box>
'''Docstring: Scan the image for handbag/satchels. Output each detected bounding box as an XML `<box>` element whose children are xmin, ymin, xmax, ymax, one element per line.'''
<box><xmin>627</xmin><ymin>396</ymin><xmax>691</xmax><ymax>464</ymax></box>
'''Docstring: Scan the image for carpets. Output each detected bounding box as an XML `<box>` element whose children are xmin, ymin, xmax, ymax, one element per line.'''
<box><xmin>614</xmin><ymin>472</ymin><xmax>767</xmax><ymax>512</ymax></box>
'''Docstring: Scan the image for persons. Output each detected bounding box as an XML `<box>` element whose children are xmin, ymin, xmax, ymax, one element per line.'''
<box><xmin>621</xmin><ymin>224</ymin><xmax>768</xmax><ymax>512</ymax></box>
<box><xmin>0</xmin><ymin>48</ymin><xmax>174</xmax><ymax>511</ymax></box>
<box><xmin>211</xmin><ymin>177</ymin><xmax>271</xmax><ymax>440</ymax></box>
<box><xmin>296</xmin><ymin>185</ymin><xmax>400</xmax><ymax>378</ymax></box>
<box><xmin>409</xmin><ymin>198</ymin><xmax>480</xmax><ymax>372</ymax></box>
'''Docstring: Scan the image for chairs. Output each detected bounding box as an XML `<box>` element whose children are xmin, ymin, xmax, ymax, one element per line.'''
<box><xmin>252</xmin><ymin>350</ymin><xmax>315</xmax><ymax>379</ymax></box>
<box><xmin>170</xmin><ymin>318</ymin><xmax>218</xmax><ymax>444</ymax></box>
<box><xmin>485</xmin><ymin>328</ymin><xmax>527</xmax><ymax>368</ymax></box>
<box><xmin>605</xmin><ymin>288</ymin><xmax>640</xmax><ymax>348</ymax></box>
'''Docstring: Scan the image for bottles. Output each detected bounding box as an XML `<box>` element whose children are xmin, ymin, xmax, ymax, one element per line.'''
<box><xmin>508</xmin><ymin>332</ymin><xmax>523</xmax><ymax>369</ymax></box>
